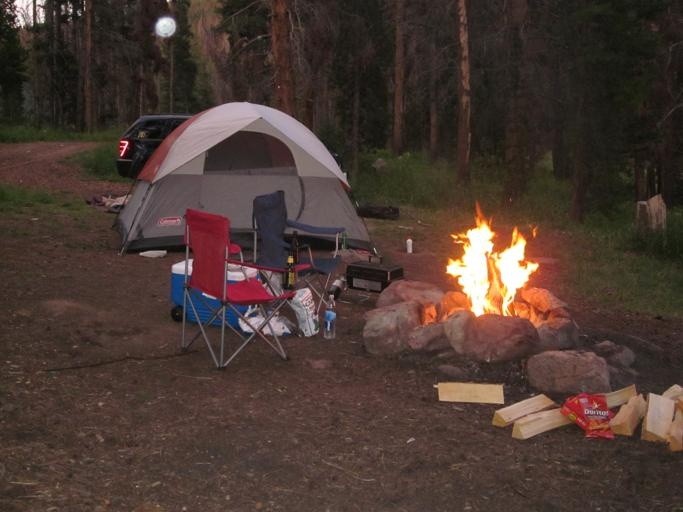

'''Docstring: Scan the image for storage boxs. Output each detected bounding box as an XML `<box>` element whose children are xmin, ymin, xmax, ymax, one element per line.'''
<box><xmin>346</xmin><ymin>262</ymin><xmax>404</xmax><ymax>293</ymax></box>
<box><xmin>171</xmin><ymin>258</ymin><xmax>262</xmax><ymax>333</ymax></box>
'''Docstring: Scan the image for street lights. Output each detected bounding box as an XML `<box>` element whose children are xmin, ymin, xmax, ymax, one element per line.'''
<box><xmin>154</xmin><ymin>17</ymin><xmax>176</xmax><ymax>112</ymax></box>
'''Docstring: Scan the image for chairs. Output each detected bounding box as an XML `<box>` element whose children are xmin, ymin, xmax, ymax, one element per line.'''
<box><xmin>180</xmin><ymin>190</ymin><xmax>343</xmax><ymax>371</ymax></box>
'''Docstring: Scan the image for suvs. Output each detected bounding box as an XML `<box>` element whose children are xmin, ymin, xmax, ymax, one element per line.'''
<box><xmin>115</xmin><ymin>113</ymin><xmax>197</xmax><ymax>180</ymax></box>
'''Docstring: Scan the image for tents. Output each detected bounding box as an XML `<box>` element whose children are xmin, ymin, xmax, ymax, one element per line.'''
<box><xmin>107</xmin><ymin>101</ymin><xmax>383</xmax><ymax>265</ymax></box>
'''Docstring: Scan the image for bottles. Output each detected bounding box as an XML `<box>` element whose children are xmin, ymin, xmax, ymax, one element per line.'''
<box><xmin>282</xmin><ymin>249</ymin><xmax>296</xmax><ymax>290</ymax></box>
<box><xmin>406</xmin><ymin>238</ymin><xmax>413</xmax><ymax>254</ymax></box>
<box><xmin>323</xmin><ymin>295</ymin><xmax>337</xmax><ymax>340</ymax></box>
